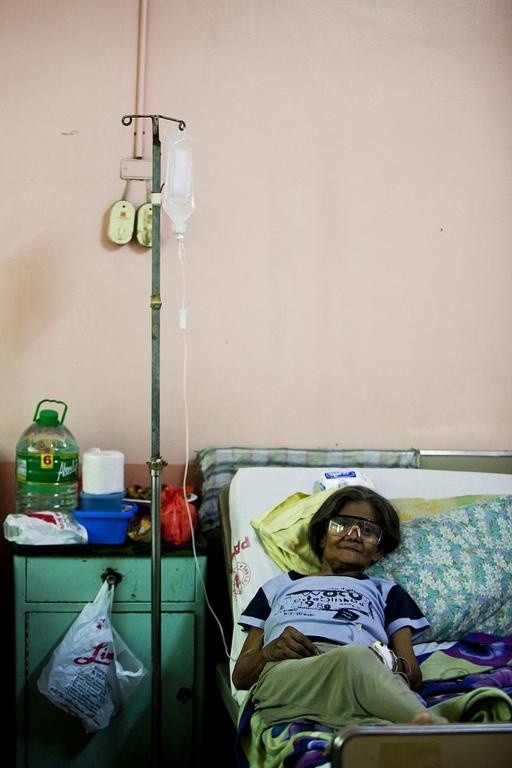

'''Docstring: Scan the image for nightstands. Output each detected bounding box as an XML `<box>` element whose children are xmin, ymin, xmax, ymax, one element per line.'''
<box><xmin>7</xmin><ymin>540</ymin><xmax>217</xmax><ymax>767</ymax></box>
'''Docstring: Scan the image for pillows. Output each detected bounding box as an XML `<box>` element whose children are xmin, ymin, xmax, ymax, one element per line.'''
<box><xmin>363</xmin><ymin>496</ymin><xmax>512</xmax><ymax>643</ymax></box>
<box><xmin>196</xmin><ymin>445</ymin><xmax>423</xmax><ymax>531</ymax></box>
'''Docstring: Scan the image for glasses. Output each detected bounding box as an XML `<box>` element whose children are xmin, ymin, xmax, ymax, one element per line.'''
<box><xmin>329</xmin><ymin>514</ymin><xmax>383</xmax><ymax>545</ymax></box>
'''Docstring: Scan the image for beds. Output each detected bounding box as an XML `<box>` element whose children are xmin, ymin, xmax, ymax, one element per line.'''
<box><xmin>197</xmin><ymin>448</ymin><xmax>512</xmax><ymax>767</ymax></box>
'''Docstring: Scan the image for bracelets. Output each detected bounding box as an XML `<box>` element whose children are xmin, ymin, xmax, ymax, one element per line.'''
<box><xmin>399</xmin><ymin>658</ymin><xmax>412</xmax><ymax>674</ymax></box>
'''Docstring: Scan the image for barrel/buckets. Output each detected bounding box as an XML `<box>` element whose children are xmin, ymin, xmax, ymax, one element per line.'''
<box><xmin>13</xmin><ymin>398</ymin><xmax>80</xmax><ymax>514</ymax></box>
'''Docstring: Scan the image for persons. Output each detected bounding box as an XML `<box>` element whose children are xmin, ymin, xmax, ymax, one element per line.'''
<box><xmin>231</xmin><ymin>484</ymin><xmax>450</xmax><ymax>727</ymax></box>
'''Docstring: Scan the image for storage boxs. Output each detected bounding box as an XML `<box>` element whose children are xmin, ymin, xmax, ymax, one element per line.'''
<box><xmin>74</xmin><ymin>503</ymin><xmax>138</xmax><ymax>542</ymax></box>
